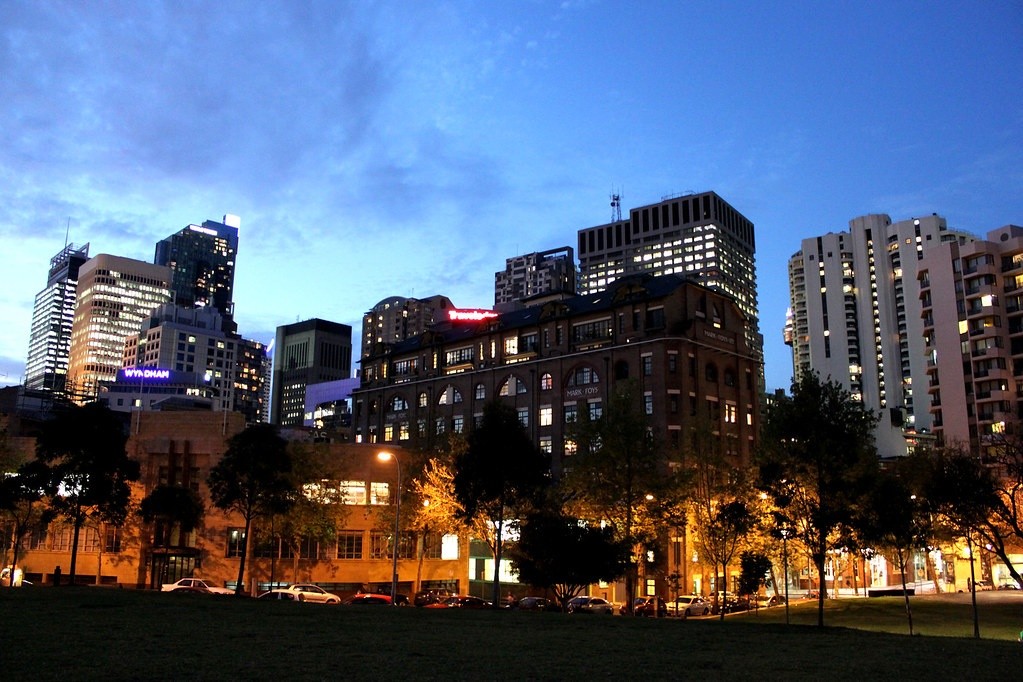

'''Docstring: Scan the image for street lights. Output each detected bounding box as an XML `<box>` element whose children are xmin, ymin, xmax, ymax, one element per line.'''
<box><xmin>376</xmin><ymin>452</ymin><xmax>402</xmax><ymax>605</ymax></box>
<box><xmin>780</xmin><ymin>529</ymin><xmax>791</xmax><ymax>623</ymax></box>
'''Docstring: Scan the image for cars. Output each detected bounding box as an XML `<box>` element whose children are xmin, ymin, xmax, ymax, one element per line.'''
<box><xmin>568</xmin><ymin>596</ymin><xmax>616</xmax><ymax>614</ymax></box>
<box><xmin>709</xmin><ymin>591</ymin><xmax>786</xmax><ymax>613</ymax></box>
<box><xmin>509</xmin><ymin>598</ymin><xmax>559</xmax><ymax>609</ymax></box>
<box><xmin>274</xmin><ymin>583</ymin><xmax>341</xmax><ymax>604</ymax></box>
<box><xmin>344</xmin><ymin>593</ymin><xmax>509</xmax><ymax>607</ymax></box>
<box><xmin>976</xmin><ymin>581</ymin><xmax>1017</xmax><ymax>591</ymax></box>
<box><xmin>620</xmin><ymin>596</ymin><xmax>666</xmax><ymax>617</ymax></box>
<box><xmin>259</xmin><ymin>591</ymin><xmax>308</xmax><ymax>602</ymax></box>
<box><xmin>0</xmin><ymin>568</ymin><xmax>32</xmax><ymax>586</ymax></box>
<box><xmin>797</xmin><ymin>591</ymin><xmax>827</xmax><ymax>602</ymax></box>
<box><xmin>666</xmin><ymin>594</ymin><xmax>712</xmax><ymax>617</ymax></box>
<box><xmin>161</xmin><ymin>578</ymin><xmax>235</xmax><ymax>595</ymax></box>
